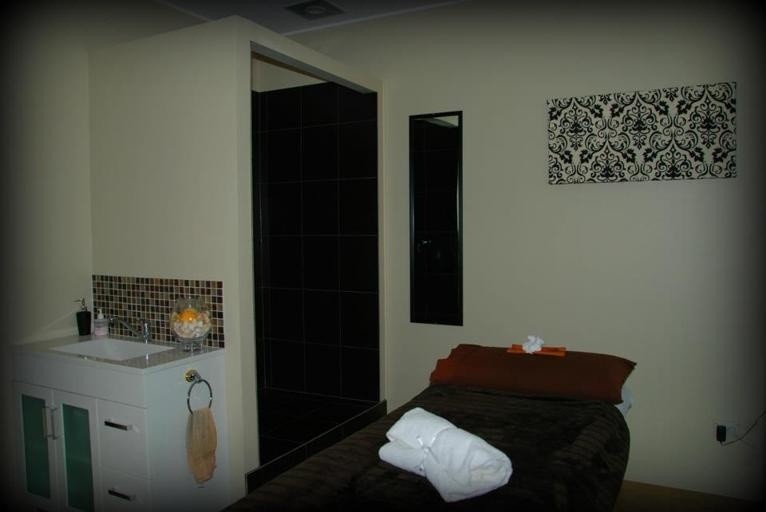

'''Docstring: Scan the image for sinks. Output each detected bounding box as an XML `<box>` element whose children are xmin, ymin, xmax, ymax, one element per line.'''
<box><xmin>50</xmin><ymin>336</ymin><xmax>176</xmax><ymax>362</ymax></box>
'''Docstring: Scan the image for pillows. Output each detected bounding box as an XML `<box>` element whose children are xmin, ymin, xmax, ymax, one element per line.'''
<box><xmin>430</xmin><ymin>343</ymin><xmax>637</xmax><ymax>405</ymax></box>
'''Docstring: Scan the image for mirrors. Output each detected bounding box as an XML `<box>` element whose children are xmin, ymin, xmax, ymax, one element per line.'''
<box><xmin>409</xmin><ymin>108</ymin><xmax>465</xmax><ymax>327</ymax></box>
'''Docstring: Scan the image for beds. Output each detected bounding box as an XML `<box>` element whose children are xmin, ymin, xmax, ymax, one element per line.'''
<box><xmin>213</xmin><ymin>342</ymin><xmax>638</xmax><ymax>510</ymax></box>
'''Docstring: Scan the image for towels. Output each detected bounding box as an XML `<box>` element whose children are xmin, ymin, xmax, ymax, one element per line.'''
<box><xmin>379</xmin><ymin>406</ymin><xmax>514</xmax><ymax>502</ymax></box>
<box><xmin>186</xmin><ymin>408</ymin><xmax>219</xmax><ymax>485</ymax></box>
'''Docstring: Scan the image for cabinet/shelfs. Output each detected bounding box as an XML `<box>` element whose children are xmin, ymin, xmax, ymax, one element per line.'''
<box><xmin>0</xmin><ymin>351</ymin><xmax>229</xmax><ymax>512</ymax></box>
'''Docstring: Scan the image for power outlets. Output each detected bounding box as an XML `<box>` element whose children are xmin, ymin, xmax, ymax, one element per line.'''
<box><xmin>714</xmin><ymin>424</ymin><xmax>737</xmax><ymax>447</ymax></box>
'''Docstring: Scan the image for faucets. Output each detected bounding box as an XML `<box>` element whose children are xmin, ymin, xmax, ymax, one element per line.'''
<box><xmin>108</xmin><ymin>315</ymin><xmax>150</xmax><ymax>343</ymax></box>
